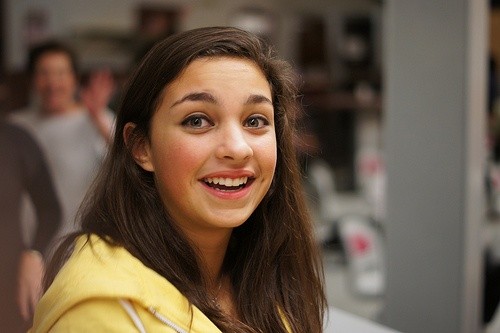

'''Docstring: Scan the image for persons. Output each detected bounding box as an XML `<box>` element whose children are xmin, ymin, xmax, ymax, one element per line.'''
<box><xmin>29</xmin><ymin>25</ymin><xmax>329</xmax><ymax>333</ymax></box>
<box><xmin>9</xmin><ymin>37</ymin><xmax>121</xmax><ymax>243</ymax></box>
<box><xmin>2</xmin><ymin>118</ymin><xmax>62</xmax><ymax>332</ymax></box>
